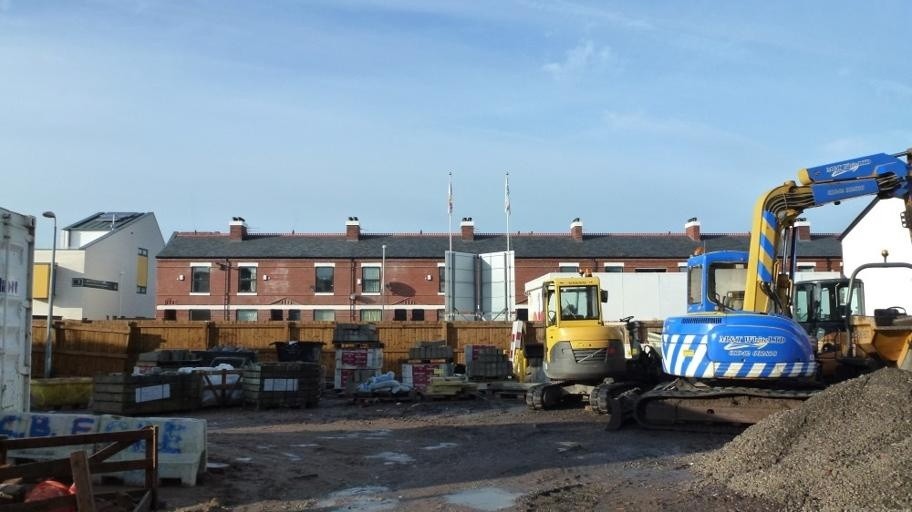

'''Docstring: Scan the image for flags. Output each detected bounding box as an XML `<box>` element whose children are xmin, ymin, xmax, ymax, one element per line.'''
<box><xmin>505</xmin><ymin>180</ymin><xmax>511</xmax><ymax>218</ymax></box>
<box><xmin>448</xmin><ymin>182</ymin><xmax>453</xmax><ymax>215</ymax></box>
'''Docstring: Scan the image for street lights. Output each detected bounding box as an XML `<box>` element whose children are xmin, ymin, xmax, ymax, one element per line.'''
<box><xmin>381</xmin><ymin>244</ymin><xmax>388</xmax><ymax>322</ymax></box>
<box><xmin>41</xmin><ymin>209</ymin><xmax>57</xmax><ymax>377</ymax></box>
